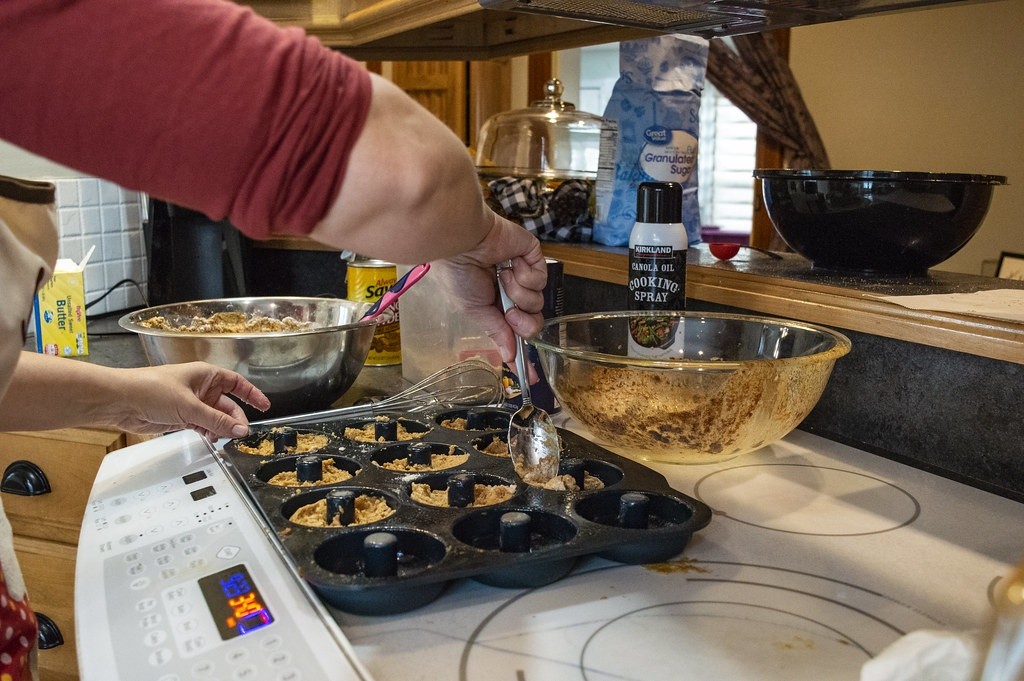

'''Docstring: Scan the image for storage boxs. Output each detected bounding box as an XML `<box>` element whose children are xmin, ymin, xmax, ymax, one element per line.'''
<box><xmin>32</xmin><ymin>245</ymin><xmax>95</xmax><ymax>357</ymax></box>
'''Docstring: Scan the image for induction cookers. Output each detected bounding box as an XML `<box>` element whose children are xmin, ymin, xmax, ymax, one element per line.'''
<box><xmin>74</xmin><ymin>408</ymin><xmax>1024</xmax><ymax>681</ymax></box>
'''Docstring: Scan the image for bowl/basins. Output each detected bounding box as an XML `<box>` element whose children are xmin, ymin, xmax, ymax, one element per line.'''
<box><xmin>752</xmin><ymin>170</ymin><xmax>1007</xmax><ymax>277</ymax></box>
<box><xmin>526</xmin><ymin>310</ymin><xmax>852</xmax><ymax>464</ymax></box>
<box><xmin>119</xmin><ymin>296</ymin><xmax>377</xmax><ymax>422</ymax></box>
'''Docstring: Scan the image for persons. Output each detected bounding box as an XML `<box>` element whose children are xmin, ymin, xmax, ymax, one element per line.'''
<box><xmin>0</xmin><ymin>0</ymin><xmax>544</xmax><ymax>681</ymax></box>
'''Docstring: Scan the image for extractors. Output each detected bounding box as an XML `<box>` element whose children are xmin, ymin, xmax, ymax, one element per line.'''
<box><xmin>477</xmin><ymin>0</ymin><xmax>996</xmax><ymax>39</ymax></box>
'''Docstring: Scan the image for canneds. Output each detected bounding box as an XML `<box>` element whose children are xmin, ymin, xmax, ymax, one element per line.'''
<box><xmin>346</xmin><ymin>257</ymin><xmax>401</xmax><ymax>367</ymax></box>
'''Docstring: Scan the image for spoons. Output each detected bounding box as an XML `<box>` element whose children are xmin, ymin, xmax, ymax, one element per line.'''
<box><xmin>496</xmin><ymin>258</ymin><xmax>558</xmax><ymax>480</ymax></box>
<box><xmin>709</xmin><ymin>242</ymin><xmax>783</xmax><ymax>261</ymax></box>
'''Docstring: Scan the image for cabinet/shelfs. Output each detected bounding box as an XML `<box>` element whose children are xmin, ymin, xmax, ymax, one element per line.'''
<box><xmin>0</xmin><ymin>423</ymin><xmax>128</xmax><ymax>681</ymax></box>
<box><xmin>392</xmin><ymin>56</ymin><xmax>513</xmax><ymax>152</ymax></box>
